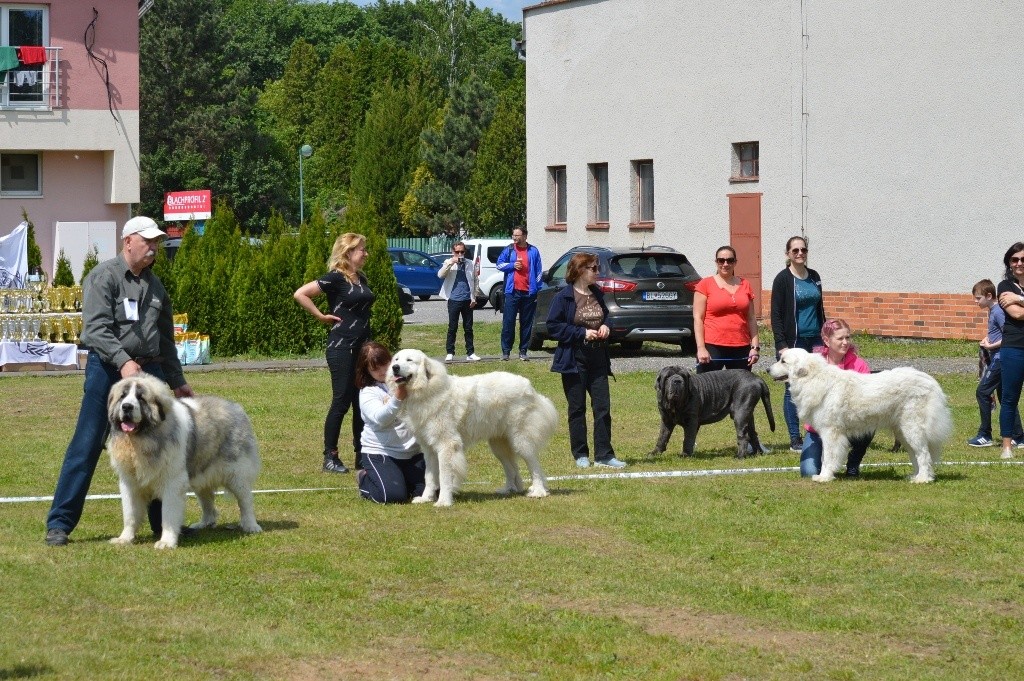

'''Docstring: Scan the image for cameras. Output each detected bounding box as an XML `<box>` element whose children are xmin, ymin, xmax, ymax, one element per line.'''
<box><xmin>458</xmin><ymin>257</ymin><xmax>465</xmax><ymax>262</ymax></box>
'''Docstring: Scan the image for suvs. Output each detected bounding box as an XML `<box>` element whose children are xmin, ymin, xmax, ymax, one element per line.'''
<box><xmin>458</xmin><ymin>238</ymin><xmax>515</xmax><ymax>308</ymax></box>
<box><xmin>530</xmin><ymin>244</ymin><xmax>702</xmax><ymax>358</ymax></box>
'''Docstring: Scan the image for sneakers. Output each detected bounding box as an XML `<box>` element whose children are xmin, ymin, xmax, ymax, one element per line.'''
<box><xmin>323</xmin><ymin>456</ymin><xmax>349</xmax><ymax>473</ymax></box>
<box><xmin>355</xmin><ymin>457</ymin><xmax>365</xmax><ymax>469</ymax></box>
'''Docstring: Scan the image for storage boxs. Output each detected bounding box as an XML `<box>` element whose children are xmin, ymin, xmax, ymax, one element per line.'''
<box><xmin>0</xmin><ymin>342</ymin><xmax>88</xmax><ymax>371</ymax></box>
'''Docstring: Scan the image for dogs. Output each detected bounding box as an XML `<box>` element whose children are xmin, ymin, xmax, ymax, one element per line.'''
<box><xmin>764</xmin><ymin>346</ymin><xmax>958</xmax><ymax>485</ymax></box>
<box><xmin>384</xmin><ymin>347</ymin><xmax>561</xmax><ymax>509</ymax></box>
<box><xmin>103</xmin><ymin>368</ymin><xmax>266</xmax><ymax>551</ymax></box>
<box><xmin>643</xmin><ymin>363</ymin><xmax>776</xmax><ymax>462</ymax></box>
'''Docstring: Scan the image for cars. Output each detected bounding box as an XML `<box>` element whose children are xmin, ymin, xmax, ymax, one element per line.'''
<box><xmin>416</xmin><ymin>252</ymin><xmax>455</xmax><ymax>268</ymax></box>
<box><xmin>383</xmin><ymin>247</ymin><xmax>447</xmax><ymax>301</ymax></box>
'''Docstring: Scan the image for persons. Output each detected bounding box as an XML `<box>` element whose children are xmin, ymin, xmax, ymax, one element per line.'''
<box><xmin>771</xmin><ymin>236</ymin><xmax>826</xmax><ymax>452</ymax></box>
<box><xmin>496</xmin><ymin>225</ymin><xmax>543</xmax><ymax>361</ymax></box>
<box><xmin>438</xmin><ymin>242</ymin><xmax>481</xmax><ymax>361</ymax></box>
<box><xmin>800</xmin><ymin>319</ymin><xmax>875</xmax><ymax>477</ymax></box>
<box><xmin>294</xmin><ymin>232</ymin><xmax>377</xmax><ymax>472</ymax></box>
<box><xmin>967</xmin><ymin>279</ymin><xmax>1024</xmax><ymax>448</ymax></box>
<box><xmin>997</xmin><ymin>242</ymin><xmax>1024</xmax><ymax>459</ymax></box>
<box><xmin>546</xmin><ymin>253</ymin><xmax>627</xmax><ymax>470</ymax></box>
<box><xmin>355</xmin><ymin>342</ymin><xmax>426</xmax><ymax>504</ymax></box>
<box><xmin>44</xmin><ymin>216</ymin><xmax>194</xmax><ymax>545</ymax></box>
<box><xmin>693</xmin><ymin>246</ymin><xmax>772</xmax><ymax>454</ymax></box>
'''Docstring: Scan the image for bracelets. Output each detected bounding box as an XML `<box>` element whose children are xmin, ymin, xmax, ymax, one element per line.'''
<box><xmin>751</xmin><ymin>346</ymin><xmax>760</xmax><ymax>351</ymax></box>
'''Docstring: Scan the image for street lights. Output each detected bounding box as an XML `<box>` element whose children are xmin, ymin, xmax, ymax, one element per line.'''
<box><xmin>299</xmin><ymin>145</ymin><xmax>313</xmax><ymax>227</ymax></box>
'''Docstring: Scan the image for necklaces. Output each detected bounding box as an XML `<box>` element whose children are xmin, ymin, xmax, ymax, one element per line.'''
<box><xmin>575</xmin><ymin>286</ymin><xmax>589</xmax><ymax>300</ymax></box>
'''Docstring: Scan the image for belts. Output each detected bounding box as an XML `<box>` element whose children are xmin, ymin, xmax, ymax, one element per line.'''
<box><xmin>575</xmin><ymin>341</ymin><xmax>609</xmax><ymax>348</ymax></box>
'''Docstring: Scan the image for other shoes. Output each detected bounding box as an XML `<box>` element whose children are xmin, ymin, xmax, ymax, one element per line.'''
<box><xmin>966</xmin><ymin>432</ymin><xmax>993</xmax><ymax>447</ymax></box>
<box><xmin>466</xmin><ymin>353</ymin><xmax>481</xmax><ymax>361</ymax></box>
<box><xmin>789</xmin><ymin>437</ymin><xmax>804</xmax><ymax>453</ymax></box>
<box><xmin>576</xmin><ymin>456</ymin><xmax>590</xmax><ymax>467</ymax></box>
<box><xmin>500</xmin><ymin>355</ymin><xmax>509</xmax><ymax>361</ymax></box>
<box><xmin>518</xmin><ymin>352</ymin><xmax>529</xmax><ymax>361</ymax></box>
<box><xmin>47</xmin><ymin>528</ymin><xmax>68</xmax><ymax>546</ymax></box>
<box><xmin>356</xmin><ymin>469</ymin><xmax>371</xmax><ymax>501</ymax></box>
<box><xmin>445</xmin><ymin>353</ymin><xmax>454</xmax><ymax>361</ymax></box>
<box><xmin>154</xmin><ymin>526</ymin><xmax>201</xmax><ymax>538</ymax></box>
<box><xmin>1000</xmin><ymin>446</ymin><xmax>1014</xmax><ymax>460</ymax></box>
<box><xmin>1000</xmin><ymin>439</ymin><xmax>1024</xmax><ymax>449</ymax></box>
<box><xmin>594</xmin><ymin>457</ymin><xmax>627</xmax><ymax>469</ymax></box>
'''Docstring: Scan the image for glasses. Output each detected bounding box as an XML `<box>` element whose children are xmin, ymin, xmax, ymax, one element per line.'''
<box><xmin>454</xmin><ymin>250</ymin><xmax>465</xmax><ymax>254</ymax></box>
<box><xmin>716</xmin><ymin>257</ymin><xmax>736</xmax><ymax>265</ymax></box>
<box><xmin>1010</xmin><ymin>257</ymin><xmax>1024</xmax><ymax>263</ymax></box>
<box><xmin>586</xmin><ymin>265</ymin><xmax>602</xmax><ymax>272</ymax></box>
<box><xmin>788</xmin><ymin>247</ymin><xmax>808</xmax><ymax>254</ymax></box>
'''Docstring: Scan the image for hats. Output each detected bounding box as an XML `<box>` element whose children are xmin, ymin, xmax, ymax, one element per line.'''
<box><xmin>120</xmin><ymin>216</ymin><xmax>169</xmax><ymax>242</ymax></box>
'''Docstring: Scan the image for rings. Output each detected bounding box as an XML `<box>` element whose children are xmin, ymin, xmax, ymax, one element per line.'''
<box><xmin>592</xmin><ymin>338</ymin><xmax>594</xmax><ymax>340</ymax></box>
<box><xmin>1003</xmin><ymin>302</ymin><xmax>1005</xmax><ymax>304</ymax></box>
